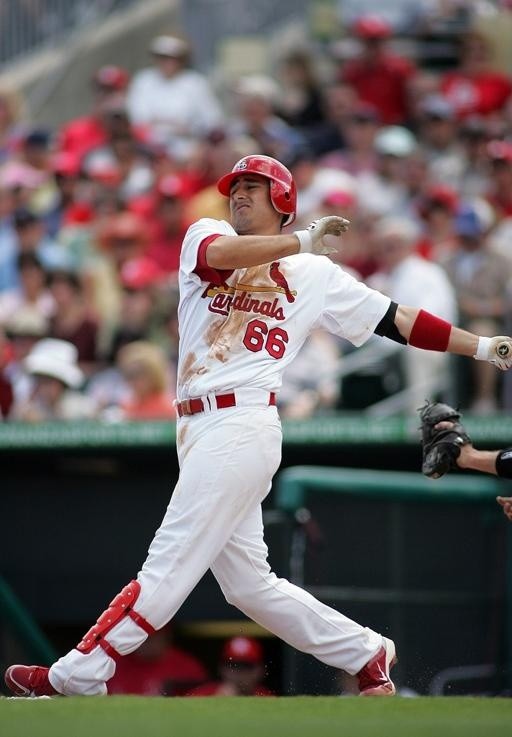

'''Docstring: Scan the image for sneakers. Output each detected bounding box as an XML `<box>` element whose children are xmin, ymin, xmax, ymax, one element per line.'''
<box><xmin>5</xmin><ymin>664</ymin><xmax>57</xmax><ymax>697</ymax></box>
<box><xmin>358</xmin><ymin>637</ymin><xmax>397</xmax><ymax>696</ymax></box>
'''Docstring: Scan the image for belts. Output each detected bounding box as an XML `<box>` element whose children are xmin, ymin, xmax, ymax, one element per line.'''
<box><xmin>177</xmin><ymin>392</ymin><xmax>276</xmax><ymax>416</ymax></box>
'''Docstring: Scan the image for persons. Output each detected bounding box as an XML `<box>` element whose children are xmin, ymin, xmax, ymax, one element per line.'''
<box><xmin>1</xmin><ymin>1</ymin><xmax>511</xmax><ymax>421</ymax></box>
<box><xmin>107</xmin><ymin>625</ymin><xmax>210</xmax><ymax>695</ymax></box>
<box><xmin>416</xmin><ymin>402</ymin><xmax>511</xmax><ymax>521</ymax></box>
<box><xmin>176</xmin><ymin>630</ymin><xmax>275</xmax><ymax>696</ymax></box>
<box><xmin>324</xmin><ymin>664</ymin><xmax>419</xmax><ymax>696</ymax></box>
<box><xmin>4</xmin><ymin>150</ymin><xmax>511</xmax><ymax>696</ymax></box>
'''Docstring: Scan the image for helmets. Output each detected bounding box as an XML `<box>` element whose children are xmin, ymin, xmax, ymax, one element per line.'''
<box><xmin>217</xmin><ymin>154</ymin><xmax>297</xmax><ymax>227</ymax></box>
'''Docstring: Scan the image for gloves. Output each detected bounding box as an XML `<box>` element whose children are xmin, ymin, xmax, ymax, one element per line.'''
<box><xmin>475</xmin><ymin>335</ymin><xmax>511</xmax><ymax>371</ymax></box>
<box><xmin>293</xmin><ymin>213</ymin><xmax>351</xmax><ymax>257</ymax></box>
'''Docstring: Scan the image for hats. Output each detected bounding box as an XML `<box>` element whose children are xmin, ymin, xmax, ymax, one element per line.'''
<box><xmin>27</xmin><ymin>338</ymin><xmax>82</xmax><ymax>388</ymax></box>
